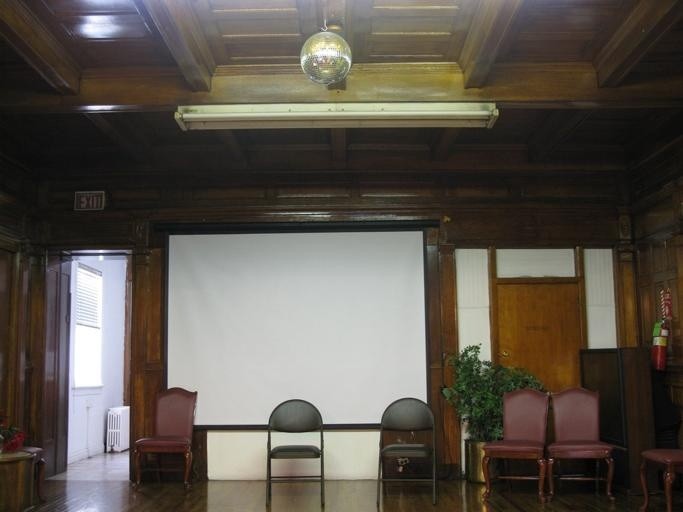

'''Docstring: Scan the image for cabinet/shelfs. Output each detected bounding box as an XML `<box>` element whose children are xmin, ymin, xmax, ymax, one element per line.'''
<box><xmin>578</xmin><ymin>345</ymin><xmax>659</xmax><ymax>497</ymax></box>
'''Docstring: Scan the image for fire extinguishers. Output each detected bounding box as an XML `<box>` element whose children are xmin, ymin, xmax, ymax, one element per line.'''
<box><xmin>650</xmin><ymin>313</ymin><xmax>670</xmax><ymax>371</ymax></box>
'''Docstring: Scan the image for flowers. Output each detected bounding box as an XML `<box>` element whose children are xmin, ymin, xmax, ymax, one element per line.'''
<box><xmin>0</xmin><ymin>407</ymin><xmax>31</xmax><ymax>455</ymax></box>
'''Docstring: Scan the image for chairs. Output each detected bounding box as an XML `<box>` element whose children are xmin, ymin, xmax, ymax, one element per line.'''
<box><xmin>545</xmin><ymin>383</ymin><xmax>617</xmax><ymax>505</ymax></box>
<box><xmin>130</xmin><ymin>386</ymin><xmax>199</xmax><ymax>495</ymax></box>
<box><xmin>480</xmin><ymin>387</ymin><xmax>548</xmax><ymax>505</ymax></box>
<box><xmin>264</xmin><ymin>397</ymin><xmax>325</xmax><ymax>507</ymax></box>
<box><xmin>375</xmin><ymin>395</ymin><xmax>436</xmax><ymax>512</ymax></box>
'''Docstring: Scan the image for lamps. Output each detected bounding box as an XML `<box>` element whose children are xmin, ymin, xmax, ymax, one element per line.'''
<box><xmin>299</xmin><ymin>30</ymin><xmax>355</xmax><ymax>89</ymax></box>
<box><xmin>172</xmin><ymin>101</ymin><xmax>499</xmax><ymax>132</ymax></box>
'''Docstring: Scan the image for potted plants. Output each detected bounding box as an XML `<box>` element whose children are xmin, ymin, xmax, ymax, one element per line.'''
<box><xmin>437</xmin><ymin>339</ymin><xmax>545</xmax><ymax>484</ymax></box>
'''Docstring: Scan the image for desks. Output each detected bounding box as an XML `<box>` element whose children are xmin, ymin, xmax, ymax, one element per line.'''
<box><xmin>0</xmin><ymin>449</ymin><xmax>37</xmax><ymax>511</ymax></box>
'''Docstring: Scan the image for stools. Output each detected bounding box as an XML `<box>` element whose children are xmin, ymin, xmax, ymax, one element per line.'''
<box><xmin>636</xmin><ymin>447</ymin><xmax>682</xmax><ymax>512</ymax></box>
<box><xmin>20</xmin><ymin>445</ymin><xmax>48</xmax><ymax>504</ymax></box>
